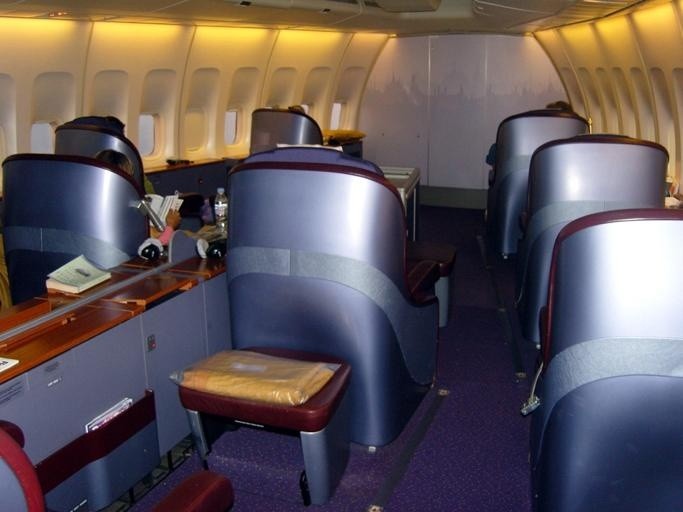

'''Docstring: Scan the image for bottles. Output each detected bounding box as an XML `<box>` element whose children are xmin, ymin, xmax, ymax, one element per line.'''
<box><xmin>214</xmin><ymin>187</ymin><xmax>227</xmax><ymax>230</ymax></box>
<box><xmin>199</xmin><ymin>198</ymin><xmax>214</xmax><ymax>226</ymax></box>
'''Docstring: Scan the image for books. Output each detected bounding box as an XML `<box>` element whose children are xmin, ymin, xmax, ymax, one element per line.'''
<box><xmin>143</xmin><ymin>194</ymin><xmax>184</xmax><ymax>228</ymax></box>
<box><xmin>45</xmin><ymin>254</ymin><xmax>112</xmax><ymax>294</ymax></box>
<box><xmin>84</xmin><ymin>397</ymin><xmax>133</xmax><ymax>433</ymax></box>
<box><xmin>0</xmin><ymin>356</ymin><xmax>19</xmax><ymax>372</ymax></box>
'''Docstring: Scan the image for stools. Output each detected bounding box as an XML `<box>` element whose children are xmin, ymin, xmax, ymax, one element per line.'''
<box><xmin>179</xmin><ymin>347</ymin><xmax>352</xmax><ymax>505</ymax></box>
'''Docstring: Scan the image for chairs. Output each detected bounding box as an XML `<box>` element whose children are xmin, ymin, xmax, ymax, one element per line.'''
<box><xmin>229</xmin><ymin>107</ymin><xmax>439</xmax><ymax>447</ymax></box>
<box><xmin>486</xmin><ymin>108</ymin><xmax>682</xmax><ymax>512</ymax></box>
<box><xmin>0</xmin><ymin>422</ymin><xmax>236</xmax><ymax>512</ymax></box>
<box><xmin>1</xmin><ymin>121</ymin><xmax>165</xmax><ymax>306</ymax></box>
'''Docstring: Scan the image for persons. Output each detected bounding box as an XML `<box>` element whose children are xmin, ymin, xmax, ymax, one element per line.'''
<box><xmin>95</xmin><ymin>149</ymin><xmax>181</xmax><ymax>247</ymax></box>
